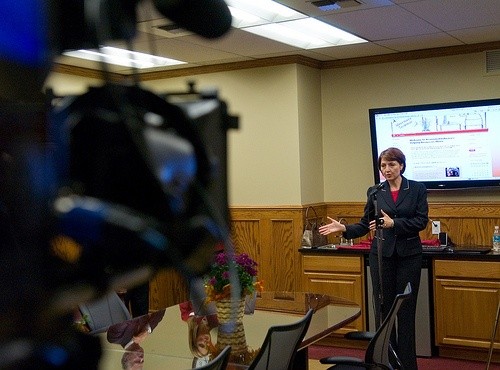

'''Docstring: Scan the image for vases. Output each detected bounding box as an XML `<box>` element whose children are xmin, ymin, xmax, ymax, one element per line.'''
<box><xmin>218</xmin><ymin>323</ymin><xmax>246</xmax><ymax>355</ymax></box>
<box><xmin>216</xmin><ymin>297</ymin><xmax>246</xmax><ymax>323</ymax></box>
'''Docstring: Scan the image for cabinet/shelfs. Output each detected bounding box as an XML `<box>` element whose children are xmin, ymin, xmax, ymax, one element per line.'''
<box><xmin>300</xmin><ymin>251</ymin><xmax>367</xmax><ymax>350</ymax></box>
<box><xmin>432</xmin><ymin>258</ymin><xmax>500</xmax><ymax>363</ymax></box>
<box><xmin>116</xmin><ymin>279</ymin><xmax>151</xmax><ymax>318</ymax></box>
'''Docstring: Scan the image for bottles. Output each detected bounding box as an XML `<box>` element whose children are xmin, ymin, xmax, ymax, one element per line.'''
<box><xmin>492</xmin><ymin>226</ymin><xmax>500</xmax><ymax>252</ymax></box>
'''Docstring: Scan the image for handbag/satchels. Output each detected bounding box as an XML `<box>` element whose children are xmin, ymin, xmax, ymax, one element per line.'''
<box><xmin>301</xmin><ymin>206</ymin><xmax>328</xmax><ymax>247</ymax></box>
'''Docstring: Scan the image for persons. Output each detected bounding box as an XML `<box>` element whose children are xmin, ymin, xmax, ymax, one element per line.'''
<box><xmin>319</xmin><ymin>147</ymin><xmax>429</xmax><ymax>370</ymax></box>
<box><xmin>107</xmin><ymin>309</ymin><xmax>166</xmax><ymax>370</ymax></box>
<box><xmin>179</xmin><ymin>301</ymin><xmax>218</xmax><ymax>358</ymax></box>
<box><xmin>122</xmin><ymin>279</ymin><xmax>149</xmax><ymax>318</ymax></box>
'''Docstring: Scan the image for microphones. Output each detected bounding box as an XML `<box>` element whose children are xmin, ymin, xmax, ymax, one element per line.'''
<box><xmin>370</xmin><ymin>183</ymin><xmax>385</xmax><ymax>197</ymax></box>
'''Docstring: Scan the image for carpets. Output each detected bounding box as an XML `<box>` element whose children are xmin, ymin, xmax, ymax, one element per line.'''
<box><xmin>305</xmin><ymin>344</ymin><xmax>500</xmax><ymax>370</ymax></box>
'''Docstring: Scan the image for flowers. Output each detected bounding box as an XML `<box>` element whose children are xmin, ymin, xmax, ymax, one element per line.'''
<box><xmin>202</xmin><ymin>240</ymin><xmax>260</xmax><ymax>308</ymax></box>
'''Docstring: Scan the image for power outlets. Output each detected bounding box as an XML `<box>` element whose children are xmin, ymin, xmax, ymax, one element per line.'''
<box><xmin>431</xmin><ymin>220</ymin><xmax>441</xmax><ymax>235</ymax></box>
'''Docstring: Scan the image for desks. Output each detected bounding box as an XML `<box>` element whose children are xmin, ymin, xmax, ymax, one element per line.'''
<box><xmin>90</xmin><ymin>289</ymin><xmax>361</xmax><ymax>370</ymax></box>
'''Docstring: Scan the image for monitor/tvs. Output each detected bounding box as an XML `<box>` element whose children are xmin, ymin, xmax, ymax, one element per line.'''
<box><xmin>368</xmin><ymin>97</ymin><xmax>500</xmax><ymax>190</ymax></box>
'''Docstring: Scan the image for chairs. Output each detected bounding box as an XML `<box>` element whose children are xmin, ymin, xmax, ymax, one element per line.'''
<box><xmin>78</xmin><ymin>289</ymin><xmax>131</xmax><ymax>332</ymax></box>
<box><xmin>246</xmin><ymin>307</ymin><xmax>315</xmax><ymax>370</ymax></box>
<box><xmin>320</xmin><ymin>281</ymin><xmax>413</xmax><ymax>370</ymax></box>
